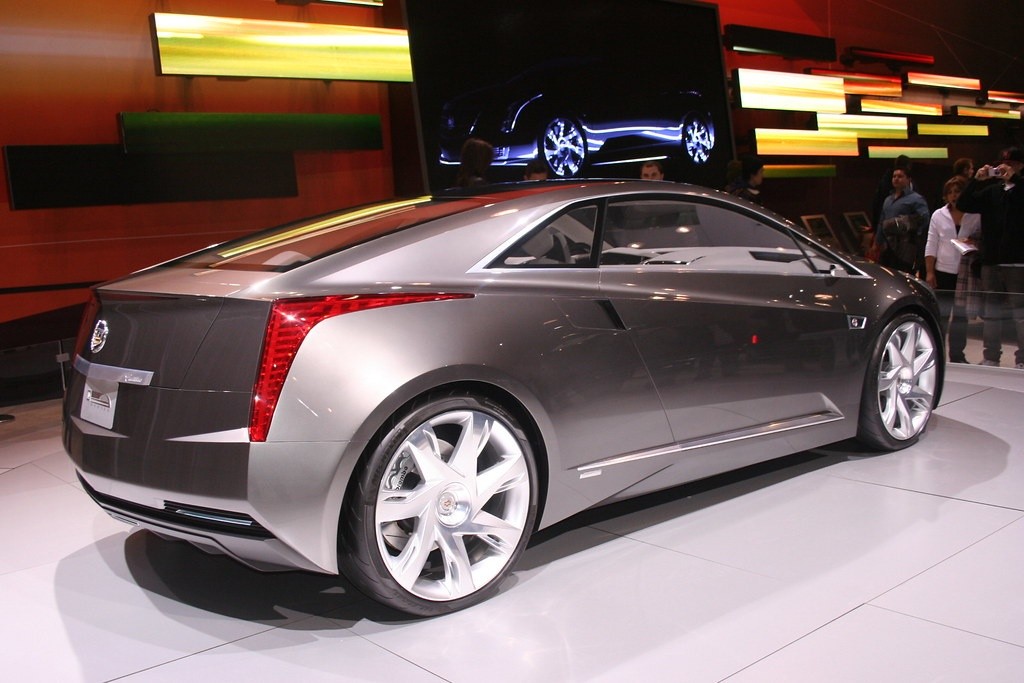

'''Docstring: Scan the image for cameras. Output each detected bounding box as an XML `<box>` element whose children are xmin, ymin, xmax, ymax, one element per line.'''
<box><xmin>989</xmin><ymin>168</ymin><xmax>1001</xmax><ymax>176</ymax></box>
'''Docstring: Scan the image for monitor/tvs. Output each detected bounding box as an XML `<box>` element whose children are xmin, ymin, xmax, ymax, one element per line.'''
<box><xmin>848</xmin><ymin>214</ymin><xmax>869</xmax><ymax>233</ymax></box>
<box><xmin>806</xmin><ymin>218</ymin><xmax>833</xmax><ymax>239</ymax></box>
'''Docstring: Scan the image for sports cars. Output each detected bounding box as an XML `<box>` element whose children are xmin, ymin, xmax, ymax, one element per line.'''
<box><xmin>60</xmin><ymin>174</ymin><xmax>951</xmax><ymax>620</ymax></box>
<box><xmin>437</xmin><ymin>74</ymin><xmax>719</xmax><ymax>180</ymax></box>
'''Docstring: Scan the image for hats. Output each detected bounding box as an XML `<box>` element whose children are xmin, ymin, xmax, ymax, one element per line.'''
<box><xmin>993</xmin><ymin>146</ymin><xmax>1024</xmax><ymax>161</ymax></box>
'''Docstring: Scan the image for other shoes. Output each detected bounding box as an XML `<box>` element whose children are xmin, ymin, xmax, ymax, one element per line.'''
<box><xmin>950</xmin><ymin>358</ymin><xmax>969</xmax><ymax>364</ymax></box>
<box><xmin>1015</xmin><ymin>363</ymin><xmax>1024</xmax><ymax>369</ymax></box>
<box><xmin>979</xmin><ymin>360</ymin><xmax>1000</xmax><ymax>366</ymax></box>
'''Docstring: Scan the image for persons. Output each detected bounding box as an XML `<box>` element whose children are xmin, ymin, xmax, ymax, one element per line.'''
<box><xmin>448</xmin><ymin>138</ymin><xmax>503</xmax><ymax>200</ymax></box>
<box><xmin>640</xmin><ymin>162</ymin><xmax>665</xmax><ymax>180</ymax></box>
<box><xmin>875</xmin><ymin>145</ymin><xmax>1024</xmax><ymax>369</ymax></box>
<box><xmin>523</xmin><ymin>161</ymin><xmax>548</xmax><ymax>180</ymax></box>
<box><xmin>724</xmin><ymin>158</ymin><xmax>767</xmax><ymax>203</ymax></box>
